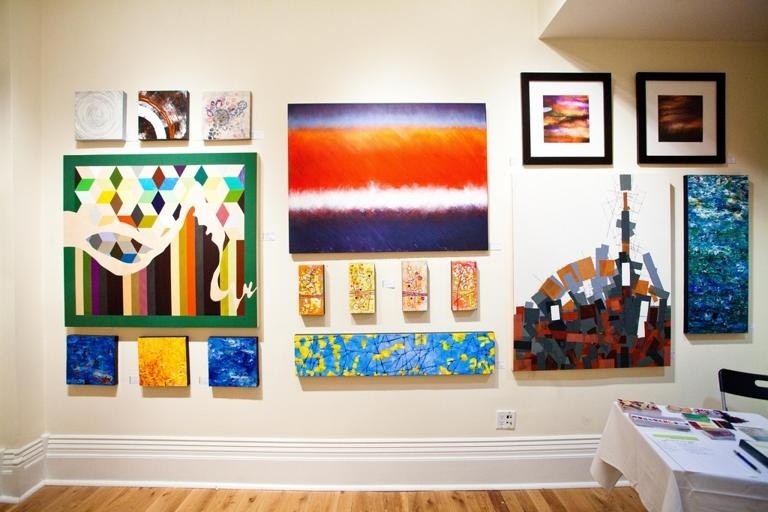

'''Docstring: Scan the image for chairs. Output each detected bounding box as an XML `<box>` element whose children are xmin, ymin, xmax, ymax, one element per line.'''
<box><xmin>718</xmin><ymin>368</ymin><xmax>767</xmax><ymax>411</ymax></box>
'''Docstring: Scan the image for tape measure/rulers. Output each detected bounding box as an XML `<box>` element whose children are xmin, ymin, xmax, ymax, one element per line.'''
<box><xmin>653</xmin><ymin>433</ymin><xmax>697</xmax><ymax>440</ymax></box>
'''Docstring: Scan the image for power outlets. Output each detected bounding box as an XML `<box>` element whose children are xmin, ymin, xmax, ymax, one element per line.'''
<box><xmin>496</xmin><ymin>411</ymin><xmax>516</xmax><ymax>429</ymax></box>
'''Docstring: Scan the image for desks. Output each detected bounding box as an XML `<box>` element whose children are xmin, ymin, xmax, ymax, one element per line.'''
<box><xmin>589</xmin><ymin>398</ymin><xmax>767</xmax><ymax>511</ymax></box>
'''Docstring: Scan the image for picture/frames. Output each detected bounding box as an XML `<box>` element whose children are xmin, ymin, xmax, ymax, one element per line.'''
<box><xmin>60</xmin><ymin>153</ymin><xmax>261</xmax><ymax>329</ymax></box>
<box><xmin>635</xmin><ymin>72</ymin><xmax>727</xmax><ymax>162</ymax></box>
<box><xmin>520</xmin><ymin>71</ymin><xmax>614</xmax><ymax>166</ymax></box>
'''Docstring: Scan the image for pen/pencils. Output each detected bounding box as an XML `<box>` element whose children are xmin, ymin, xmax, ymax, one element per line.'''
<box><xmin>733</xmin><ymin>449</ymin><xmax>761</xmax><ymax>473</ymax></box>
